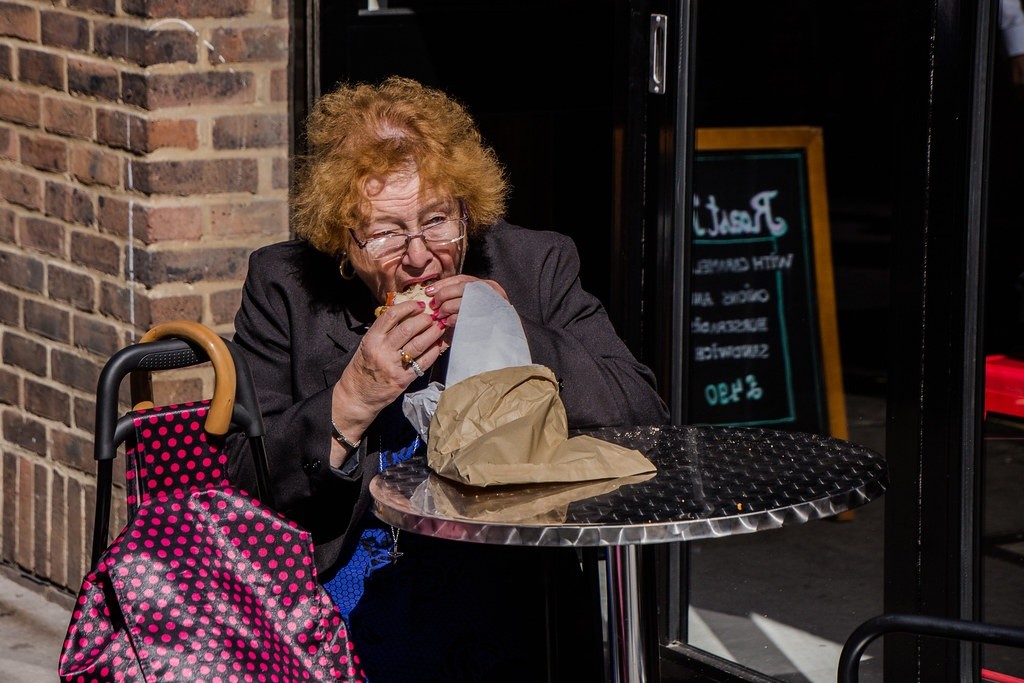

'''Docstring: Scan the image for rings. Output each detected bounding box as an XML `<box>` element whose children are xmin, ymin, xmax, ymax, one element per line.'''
<box><xmin>401</xmin><ymin>350</ymin><xmax>412</xmax><ymax>363</ymax></box>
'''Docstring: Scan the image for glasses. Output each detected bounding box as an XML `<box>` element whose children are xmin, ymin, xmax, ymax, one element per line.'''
<box><xmin>355</xmin><ymin>202</ymin><xmax>469</xmax><ymax>261</ymax></box>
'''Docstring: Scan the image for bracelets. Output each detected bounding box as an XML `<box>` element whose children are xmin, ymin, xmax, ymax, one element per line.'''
<box><xmin>331</xmin><ymin>420</ymin><xmax>362</xmax><ymax>449</ymax></box>
<box><xmin>412</xmin><ymin>362</ymin><xmax>424</xmax><ymax>377</ymax></box>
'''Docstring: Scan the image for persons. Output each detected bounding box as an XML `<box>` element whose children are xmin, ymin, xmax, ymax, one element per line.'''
<box><xmin>231</xmin><ymin>76</ymin><xmax>669</xmax><ymax>683</ymax></box>
<box><xmin>1000</xmin><ymin>0</ymin><xmax>1024</xmax><ymax>81</ymax></box>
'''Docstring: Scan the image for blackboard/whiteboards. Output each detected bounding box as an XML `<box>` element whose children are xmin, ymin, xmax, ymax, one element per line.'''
<box><xmin>614</xmin><ymin>128</ymin><xmax>847</xmax><ymax>439</ymax></box>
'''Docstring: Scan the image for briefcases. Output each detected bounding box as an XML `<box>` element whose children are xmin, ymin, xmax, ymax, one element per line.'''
<box><xmin>57</xmin><ymin>335</ymin><xmax>367</xmax><ymax>683</ymax></box>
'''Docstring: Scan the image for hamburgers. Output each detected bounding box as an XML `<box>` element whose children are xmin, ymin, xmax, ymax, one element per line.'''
<box><xmin>374</xmin><ymin>280</ymin><xmax>457</xmax><ymax>356</ymax></box>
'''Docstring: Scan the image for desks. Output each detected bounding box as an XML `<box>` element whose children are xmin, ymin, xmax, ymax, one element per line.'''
<box><xmin>370</xmin><ymin>425</ymin><xmax>889</xmax><ymax>683</ymax></box>
